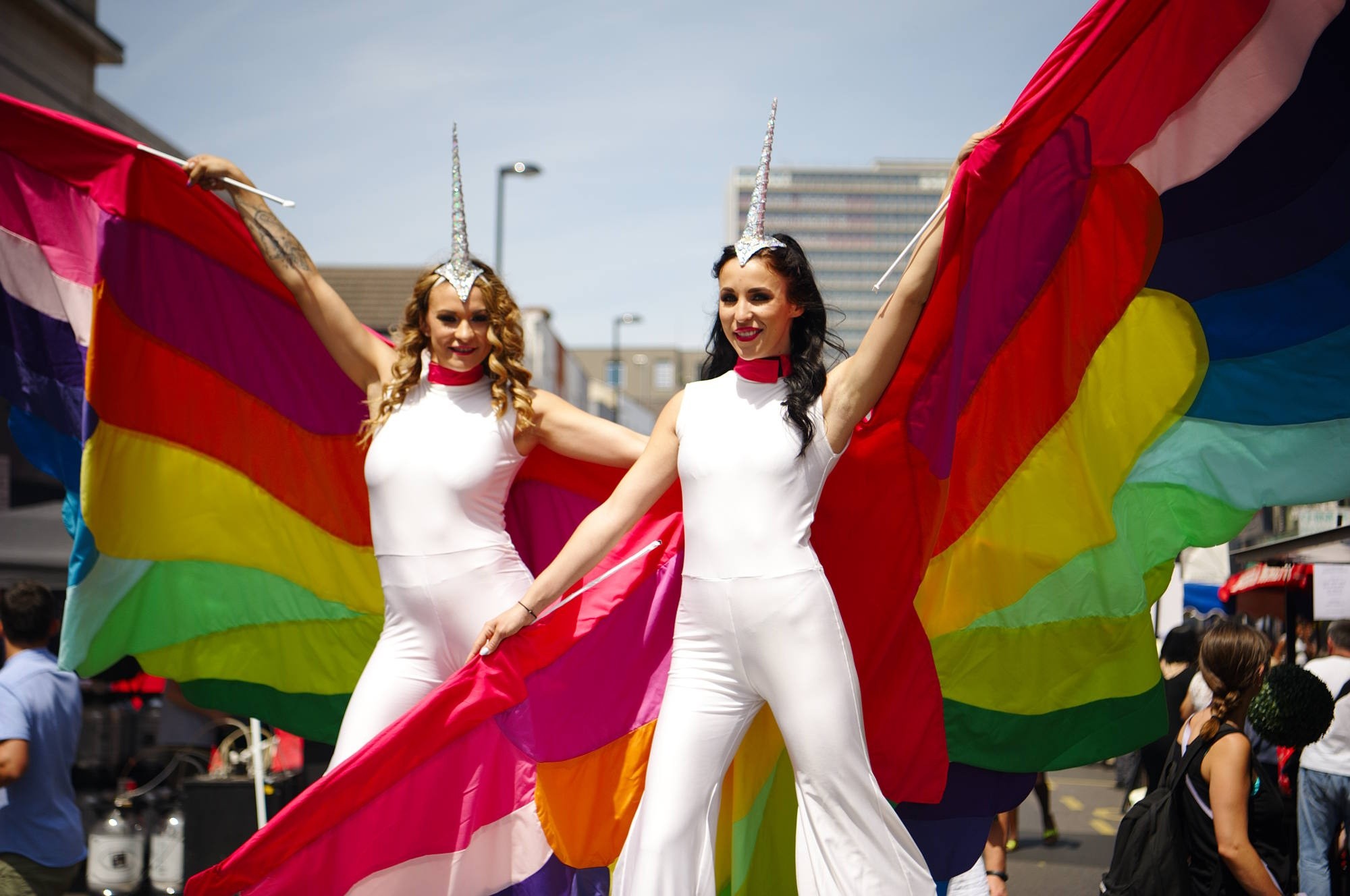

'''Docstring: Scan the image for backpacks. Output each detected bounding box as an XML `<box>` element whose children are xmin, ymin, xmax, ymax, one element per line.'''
<box><xmin>1098</xmin><ymin>716</ymin><xmax>1254</xmax><ymax>896</ymax></box>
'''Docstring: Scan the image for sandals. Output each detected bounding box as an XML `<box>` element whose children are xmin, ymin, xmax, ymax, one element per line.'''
<box><xmin>1005</xmin><ymin>840</ymin><xmax>1019</xmax><ymax>853</ymax></box>
<box><xmin>1042</xmin><ymin>826</ymin><xmax>1058</xmax><ymax>847</ymax></box>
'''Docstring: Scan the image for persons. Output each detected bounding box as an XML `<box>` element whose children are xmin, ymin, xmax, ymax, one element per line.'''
<box><xmin>466</xmin><ymin>118</ymin><xmax>1004</xmax><ymax>896</ymax></box>
<box><xmin>0</xmin><ymin>578</ymin><xmax>86</xmax><ymax>896</ymax></box>
<box><xmin>941</xmin><ymin>771</ymin><xmax>1080</xmax><ymax>896</ymax></box>
<box><xmin>183</xmin><ymin>122</ymin><xmax>651</xmax><ymax>780</ymax></box>
<box><xmin>1114</xmin><ymin>613</ymin><xmax>1350</xmax><ymax>896</ymax></box>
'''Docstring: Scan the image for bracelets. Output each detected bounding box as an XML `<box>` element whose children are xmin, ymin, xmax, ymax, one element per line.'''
<box><xmin>518</xmin><ymin>601</ymin><xmax>538</xmax><ymax>618</ymax></box>
<box><xmin>987</xmin><ymin>870</ymin><xmax>1009</xmax><ymax>882</ymax></box>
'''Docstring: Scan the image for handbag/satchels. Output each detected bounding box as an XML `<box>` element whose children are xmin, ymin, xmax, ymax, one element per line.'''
<box><xmin>1282</xmin><ymin>748</ymin><xmax>1301</xmax><ymax>798</ymax></box>
